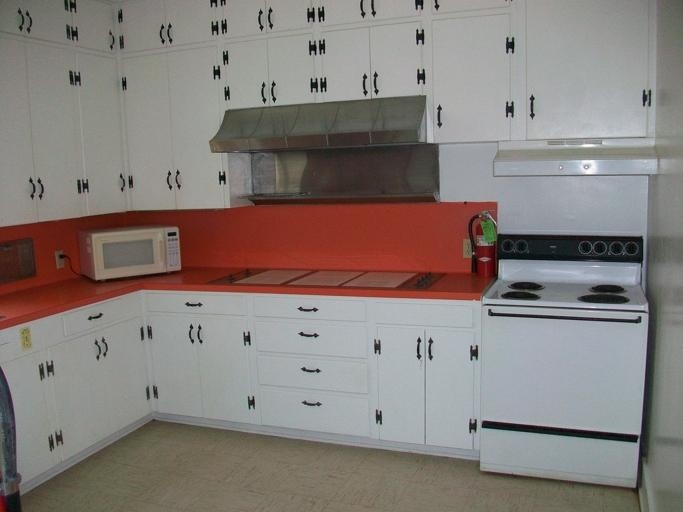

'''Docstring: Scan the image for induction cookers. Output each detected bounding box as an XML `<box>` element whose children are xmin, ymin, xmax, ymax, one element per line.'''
<box><xmin>204</xmin><ymin>266</ymin><xmax>445</xmax><ymax>292</ymax></box>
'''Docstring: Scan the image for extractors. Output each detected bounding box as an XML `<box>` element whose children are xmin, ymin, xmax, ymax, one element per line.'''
<box><xmin>209</xmin><ymin>96</ymin><xmax>428</xmax><ymax>154</ymax></box>
<box><xmin>494</xmin><ymin>138</ymin><xmax>659</xmax><ymax>177</ymax></box>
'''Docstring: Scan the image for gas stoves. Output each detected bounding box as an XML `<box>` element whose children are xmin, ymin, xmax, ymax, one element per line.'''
<box><xmin>482</xmin><ymin>278</ymin><xmax>648</xmax><ymax>313</ymax></box>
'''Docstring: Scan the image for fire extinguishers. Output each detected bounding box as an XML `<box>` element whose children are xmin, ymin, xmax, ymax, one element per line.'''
<box><xmin>468</xmin><ymin>211</ymin><xmax>497</xmax><ymax>278</ymax></box>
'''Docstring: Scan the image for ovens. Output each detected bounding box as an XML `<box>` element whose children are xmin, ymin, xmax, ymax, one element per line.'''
<box><xmin>481</xmin><ymin>309</ymin><xmax>646</xmax><ymax>492</ymax></box>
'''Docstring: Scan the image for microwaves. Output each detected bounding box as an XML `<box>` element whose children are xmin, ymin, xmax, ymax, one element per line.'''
<box><xmin>79</xmin><ymin>225</ymin><xmax>182</xmax><ymax>281</ymax></box>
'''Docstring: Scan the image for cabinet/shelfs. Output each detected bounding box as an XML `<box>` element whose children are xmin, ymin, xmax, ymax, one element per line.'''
<box><xmin>428</xmin><ymin>1</ymin><xmax>514</xmax><ymax>144</ymax></box>
<box><xmin>377</xmin><ymin>301</ymin><xmax>475</xmax><ymax>453</ymax></box>
<box><xmin>255</xmin><ymin>295</ymin><xmax>375</xmax><ymax>440</ymax></box>
<box><xmin>48</xmin><ymin>293</ymin><xmax>150</xmax><ymax>464</ymax></box>
<box><xmin>2</xmin><ymin>0</ymin><xmax>88</xmax><ymax>226</ymax></box>
<box><xmin>71</xmin><ymin>0</ymin><xmax>126</xmax><ymax>217</ymax></box>
<box><xmin>0</xmin><ymin>314</ymin><xmax>64</xmax><ymax>485</ymax></box>
<box><xmin>120</xmin><ymin>1</ymin><xmax>228</xmax><ymax>211</ymax></box>
<box><xmin>146</xmin><ymin>291</ymin><xmax>253</xmax><ymax>424</ymax></box>
<box><xmin>225</xmin><ymin>0</ymin><xmax>313</xmax><ymax>111</ymax></box>
<box><xmin>524</xmin><ymin>3</ymin><xmax>650</xmax><ymax>140</ymax></box>
<box><xmin>314</xmin><ymin>1</ymin><xmax>426</xmax><ymax>97</ymax></box>
